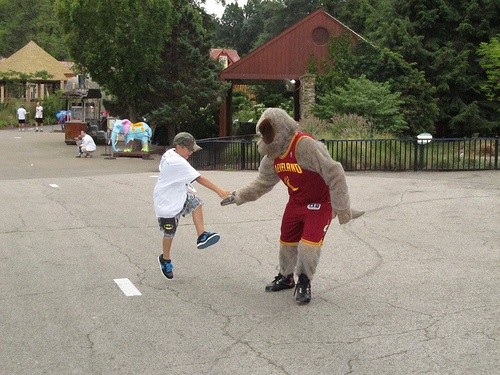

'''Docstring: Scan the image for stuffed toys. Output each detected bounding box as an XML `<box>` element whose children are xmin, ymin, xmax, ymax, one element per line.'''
<box><xmin>222</xmin><ymin>109</ymin><xmax>352</xmax><ymax>307</ymax></box>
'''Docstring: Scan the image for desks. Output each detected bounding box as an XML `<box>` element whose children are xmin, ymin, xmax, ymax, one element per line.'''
<box><xmin>65</xmin><ymin>121</ymin><xmax>87</xmax><ymax>144</ymax></box>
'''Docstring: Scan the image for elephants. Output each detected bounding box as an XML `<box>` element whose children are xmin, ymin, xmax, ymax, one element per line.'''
<box><xmin>108</xmin><ymin>119</ymin><xmax>152</xmax><ymax>152</ymax></box>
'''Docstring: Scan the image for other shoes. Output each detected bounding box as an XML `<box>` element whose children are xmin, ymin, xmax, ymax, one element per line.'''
<box><xmin>18</xmin><ymin>128</ymin><xmax>25</xmax><ymax>131</ymax></box>
<box><xmin>85</xmin><ymin>154</ymin><xmax>92</xmax><ymax>158</ymax></box>
<box><xmin>35</xmin><ymin>128</ymin><xmax>43</xmax><ymax>132</ymax></box>
<box><xmin>76</xmin><ymin>155</ymin><xmax>81</xmax><ymax>158</ymax></box>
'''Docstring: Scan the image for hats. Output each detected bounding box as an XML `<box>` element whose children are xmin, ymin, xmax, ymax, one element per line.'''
<box><xmin>173</xmin><ymin>132</ymin><xmax>202</xmax><ymax>152</ymax></box>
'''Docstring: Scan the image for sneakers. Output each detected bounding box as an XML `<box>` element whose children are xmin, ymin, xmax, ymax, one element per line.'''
<box><xmin>265</xmin><ymin>273</ymin><xmax>295</xmax><ymax>291</ymax></box>
<box><xmin>157</xmin><ymin>254</ymin><xmax>174</xmax><ymax>280</ymax></box>
<box><xmin>197</xmin><ymin>231</ymin><xmax>221</xmax><ymax>249</ymax></box>
<box><xmin>293</xmin><ymin>273</ymin><xmax>311</xmax><ymax>304</ymax></box>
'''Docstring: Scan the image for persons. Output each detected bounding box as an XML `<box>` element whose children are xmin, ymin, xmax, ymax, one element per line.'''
<box><xmin>153</xmin><ymin>133</ymin><xmax>232</xmax><ymax>280</ymax></box>
<box><xmin>16</xmin><ymin>101</ymin><xmax>97</xmax><ymax>159</ymax></box>
